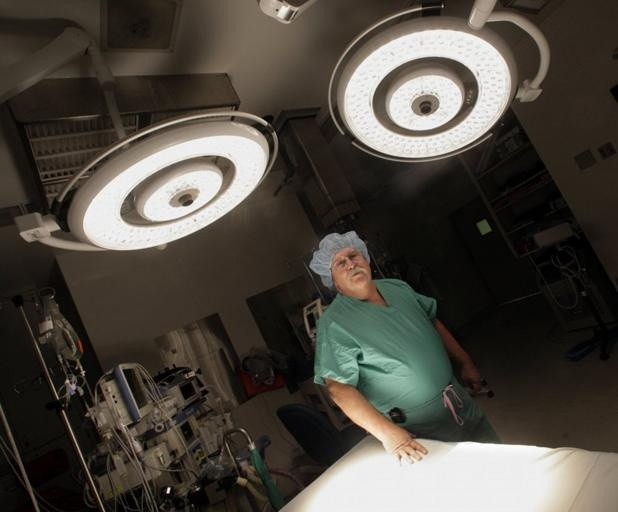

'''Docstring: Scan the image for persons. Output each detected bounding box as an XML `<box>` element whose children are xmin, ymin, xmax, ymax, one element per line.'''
<box><xmin>308</xmin><ymin>228</ymin><xmax>503</xmax><ymax>469</ymax></box>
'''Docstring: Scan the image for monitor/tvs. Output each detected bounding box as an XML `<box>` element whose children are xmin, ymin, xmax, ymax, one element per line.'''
<box><xmin>115</xmin><ymin>363</ymin><xmax>152</xmax><ymax>423</ymax></box>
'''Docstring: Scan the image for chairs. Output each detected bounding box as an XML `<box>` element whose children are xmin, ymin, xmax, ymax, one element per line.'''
<box><xmin>277</xmin><ymin>403</ymin><xmax>368</xmax><ymax>465</ymax></box>
